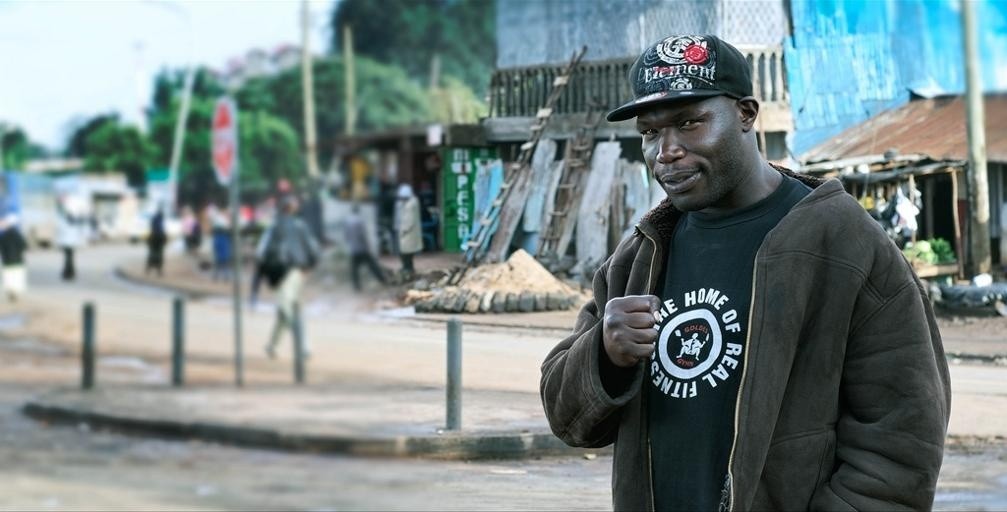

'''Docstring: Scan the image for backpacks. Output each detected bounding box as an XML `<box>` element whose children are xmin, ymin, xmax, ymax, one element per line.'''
<box><xmin>262</xmin><ymin>212</ymin><xmax>295</xmax><ymax>287</ymax></box>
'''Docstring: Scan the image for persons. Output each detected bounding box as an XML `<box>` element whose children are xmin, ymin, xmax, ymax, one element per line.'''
<box><xmin>249</xmin><ymin>190</ymin><xmax>323</xmax><ymax>361</ymax></box>
<box><xmin>206</xmin><ymin>204</ymin><xmax>232</xmax><ymax>286</ymax></box>
<box><xmin>143</xmin><ymin>200</ymin><xmax>167</xmax><ymax>280</ymax></box>
<box><xmin>396</xmin><ymin>183</ymin><xmax>425</xmax><ymax>283</ymax></box>
<box><xmin>342</xmin><ymin>203</ymin><xmax>390</xmax><ymax>296</ymax></box>
<box><xmin>538</xmin><ymin>31</ymin><xmax>954</xmax><ymax>512</ymax></box>
<box><xmin>0</xmin><ymin>213</ymin><xmax>32</xmax><ymax>305</ymax></box>
<box><xmin>56</xmin><ymin>205</ymin><xmax>81</xmax><ymax>281</ymax></box>
<box><xmin>180</xmin><ymin>203</ymin><xmax>202</xmax><ymax>278</ymax></box>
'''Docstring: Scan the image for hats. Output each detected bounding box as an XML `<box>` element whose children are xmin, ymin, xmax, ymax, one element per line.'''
<box><xmin>606</xmin><ymin>33</ymin><xmax>753</xmax><ymax>122</ymax></box>
<box><xmin>396</xmin><ymin>184</ymin><xmax>413</xmax><ymax>199</ymax></box>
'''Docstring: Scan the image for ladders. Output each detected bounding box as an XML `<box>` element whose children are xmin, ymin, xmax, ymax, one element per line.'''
<box><xmin>446</xmin><ymin>45</ymin><xmax>608</xmax><ymax>287</ymax></box>
